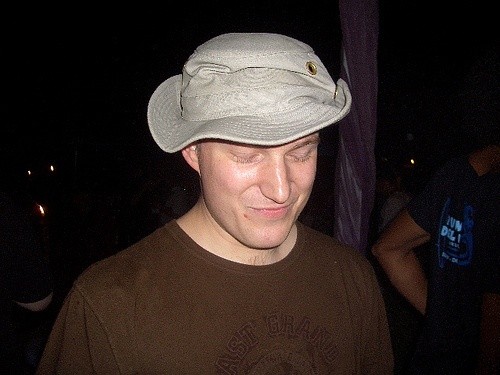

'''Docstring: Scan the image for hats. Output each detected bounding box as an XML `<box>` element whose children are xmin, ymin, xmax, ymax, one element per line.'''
<box><xmin>147</xmin><ymin>33</ymin><xmax>352</xmax><ymax>153</ymax></box>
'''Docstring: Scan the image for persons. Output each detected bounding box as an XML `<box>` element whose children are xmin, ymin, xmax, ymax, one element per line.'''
<box><xmin>34</xmin><ymin>33</ymin><xmax>393</xmax><ymax>375</ymax></box>
<box><xmin>372</xmin><ymin>141</ymin><xmax>500</xmax><ymax>375</ymax></box>
